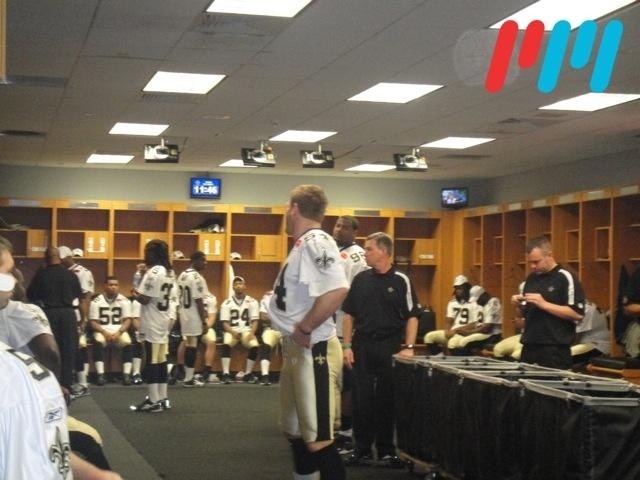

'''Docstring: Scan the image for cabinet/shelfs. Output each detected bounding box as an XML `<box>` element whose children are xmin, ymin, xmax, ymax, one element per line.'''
<box><xmin>451</xmin><ymin>183</ymin><xmax>640</xmax><ymax>359</ymax></box>
<box><xmin>1</xmin><ymin>197</ymin><xmax>452</xmax><ymax>338</ymax></box>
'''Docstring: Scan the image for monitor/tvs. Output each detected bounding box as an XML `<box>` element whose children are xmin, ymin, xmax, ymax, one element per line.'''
<box><xmin>143</xmin><ymin>143</ymin><xmax>180</xmax><ymax>163</ymax></box>
<box><xmin>300</xmin><ymin>150</ymin><xmax>334</xmax><ymax>168</ymax></box>
<box><xmin>441</xmin><ymin>187</ymin><xmax>469</xmax><ymax>210</ymax></box>
<box><xmin>241</xmin><ymin>147</ymin><xmax>276</xmax><ymax>168</ymax></box>
<box><xmin>393</xmin><ymin>154</ymin><xmax>429</xmax><ymax>172</ymax></box>
<box><xmin>190</xmin><ymin>177</ymin><xmax>222</xmax><ymax>199</ymax></box>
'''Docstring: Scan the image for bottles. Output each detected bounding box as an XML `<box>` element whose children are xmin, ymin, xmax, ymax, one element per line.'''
<box><xmin>204</xmin><ymin>239</ymin><xmax>209</xmax><ymax>254</ymax></box>
<box><xmin>424</xmin><ymin>303</ymin><xmax>432</xmax><ymax>312</ymax></box>
<box><xmin>88</xmin><ymin>236</ymin><xmax>94</xmax><ymax>253</ymax></box>
<box><xmin>132</xmin><ymin>268</ymin><xmax>142</xmax><ymax>291</ymax></box>
<box><xmin>99</xmin><ymin>236</ymin><xmax>105</xmax><ymax>252</ymax></box>
<box><xmin>215</xmin><ymin>239</ymin><xmax>221</xmax><ymax>255</ymax></box>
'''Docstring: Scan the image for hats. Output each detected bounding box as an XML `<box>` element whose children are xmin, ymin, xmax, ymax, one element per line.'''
<box><xmin>173</xmin><ymin>250</ymin><xmax>184</xmax><ymax>258</ymax></box>
<box><xmin>58</xmin><ymin>246</ymin><xmax>71</xmax><ymax>260</ymax></box>
<box><xmin>73</xmin><ymin>248</ymin><xmax>84</xmax><ymax>257</ymax></box>
<box><xmin>231</xmin><ymin>252</ymin><xmax>241</xmax><ymax>260</ymax></box>
<box><xmin>468</xmin><ymin>285</ymin><xmax>486</xmax><ymax>303</ymax></box>
<box><xmin>232</xmin><ymin>276</ymin><xmax>246</xmax><ymax>284</ymax></box>
<box><xmin>450</xmin><ymin>275</ymin><xmax>469</xmax><ymax>287</ymax></box>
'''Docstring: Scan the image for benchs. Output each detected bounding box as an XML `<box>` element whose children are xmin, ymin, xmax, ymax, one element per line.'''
<box><xmin>68</xmin><ymin>343</ymin><xmax>282</xmax><ymax>373</ymax></box>
<box><xmin>415</xmin><ymin>341</ymin><xmax>640</xmax><ymax>385</ymax></box>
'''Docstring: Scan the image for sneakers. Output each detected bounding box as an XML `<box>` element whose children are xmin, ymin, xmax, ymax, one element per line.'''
<box><xmin>73</xmin><ymin>373</ymin><xmax>271</xmax><ymax>412</ymax></box>
<box><xmin>335</xmin><ymin>433</ymin><xmax>406</xmax><ymax>470</ymax></box>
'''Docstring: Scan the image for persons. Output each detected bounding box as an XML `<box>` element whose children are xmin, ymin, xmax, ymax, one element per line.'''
<box><xmin>0</xmin><ymin>183</ymin><xmax>640</xmax><ymax>480</ymax></box>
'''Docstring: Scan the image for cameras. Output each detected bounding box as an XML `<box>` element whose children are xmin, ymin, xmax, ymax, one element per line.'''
<box><xmin>518</xmin><ymin>297</ymin><xmax>532</xmax><ymax>309</ymax></box>
